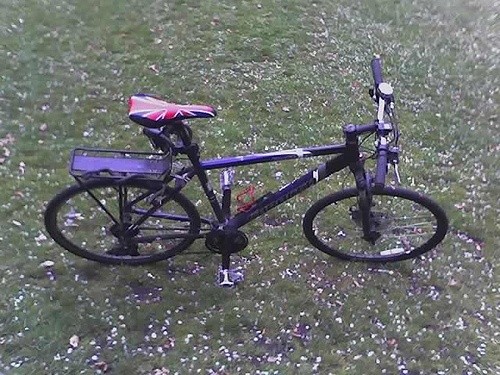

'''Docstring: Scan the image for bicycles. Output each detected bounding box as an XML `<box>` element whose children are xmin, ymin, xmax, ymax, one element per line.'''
<box><xmin>42</xmin><ymin>52</ymin><xmax>449</xmax><ymax>288</ymax></box>
